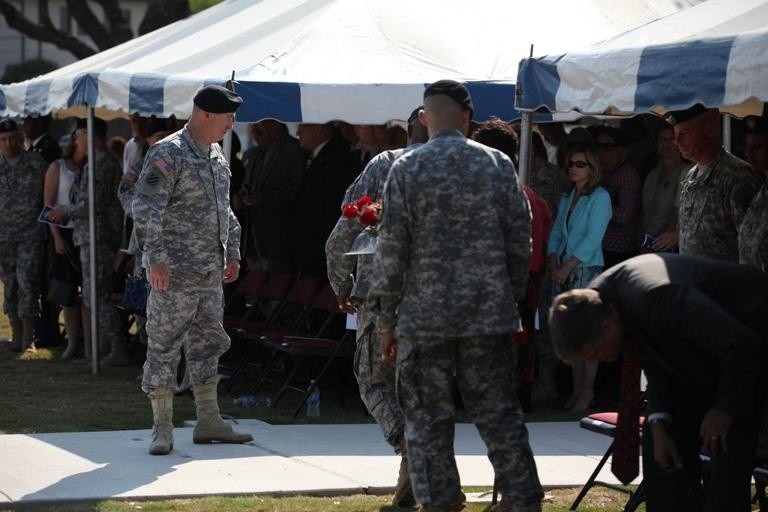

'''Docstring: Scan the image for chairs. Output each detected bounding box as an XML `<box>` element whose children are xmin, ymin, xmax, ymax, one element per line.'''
<box><xmin>57</xmin><ymin>247</ymin><xmax>136</xmax><ymax>340</ymax></box>
<box><xmin>222</xmin><ymin>272</ymin><xmax>369</xmax><ymax>418</ymax></box>
<box><xmin>571</xmin><ymin>387</ymin><xmax>649</xmax><ymax>511</ymax></box>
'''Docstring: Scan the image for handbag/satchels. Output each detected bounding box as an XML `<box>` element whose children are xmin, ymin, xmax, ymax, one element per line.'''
<box><xmin>120</xmin><ymin>275</ymin><xmax>150</xmax><ymax>317</ymax></box>
<box><xmin>47</xmin><ymin>254</ymin><xmax>81</xmax><ymax>307</ymax></box>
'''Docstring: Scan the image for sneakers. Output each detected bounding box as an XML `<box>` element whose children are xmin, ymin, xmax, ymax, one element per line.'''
<box><xmin>393</xmin><ymin>440</ymin><xmax>416</xmax><ymax>508</ymax></box>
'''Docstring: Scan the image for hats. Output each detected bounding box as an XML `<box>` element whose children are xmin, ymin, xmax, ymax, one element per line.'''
<box><xmin>742</xmin><ymin>114</ymin><xmax>767</xmax><ymax>135</ymax></box>
<box><xmin>193</xmin><ymin>85</ymin><xmax>243</xmax><ymax>113</ymax></box>
<box><xmin>77</xmin><ymin>117</ymin><xmax>106</xmax><ymax>139</ymax></box>
<box><xmin>141</xmin><ymin>116</ymin><xmax>176</xmax><ymax>139</ymax></box>
<box><xmin>423</xmin><ymin>80</ymin><xmax>474</xmax><ymax>119</ymax></box>
<box><xmin>0</xmin><ymin>119</ymin><xmax>18</xmax><ymax>132</ymax></box>
<box><xmin>664</xmin><ymin>105</ymin><xmax>708</xmax><ymax>126</ymax></box>
<box><xmin>409</xmin><ymin>106</ymin><xmax>424</xmax><ymax>121</ymax></box>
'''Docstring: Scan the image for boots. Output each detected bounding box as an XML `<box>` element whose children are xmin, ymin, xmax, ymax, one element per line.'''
<box><xmin>101</xmin><ymin>335</ymin><xmax>130</xmax><ymax>366</ymax></box>
<box><xmin>83</xmin><ymin>329</ymin><xmax>93</xmax><ymax>360</ymax></box>
<box><xmin>61</xmin><ymin>327</ymin><xmax>80</xmax><ymax>360</ymax></box>
<box><xmin>192</xmin><ymin>372</ymin><xmax>253</xmax><ymax>443</ymax></box>
<box><xmin>531</xmin><ymin>363</ymin><xmax>560</xmax><ymax>405</ymax></box>
<box><xmin>21</xmin><ymin>317</ymin><xmax>37</xmax><ymax>354</ymax></box>
<box><xmin>10</xmin><ymin>319</ymin><xmax>23</xmax><ymax>350</ymax></box>
<box><xmin>148</xmin><ymin>389</ymin><xmax>174</xmax><ymax>454</ymax></box>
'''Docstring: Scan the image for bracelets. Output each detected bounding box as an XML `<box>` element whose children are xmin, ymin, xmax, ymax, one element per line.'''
<box><xmin>649</xmin><ymin>418</ymin><xmax>668</xmax><ymax>426</ymax></box>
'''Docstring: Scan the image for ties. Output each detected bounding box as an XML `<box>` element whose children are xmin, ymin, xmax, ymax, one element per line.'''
<box><xmin>611</xmin><ymin>344</ymin><xmax>639</xmax><ymax>486</ymax></box>
<box><xmin>306</xmin><ymin>152</ymin><xmax>314</xmax><ymax>170</ymax></box>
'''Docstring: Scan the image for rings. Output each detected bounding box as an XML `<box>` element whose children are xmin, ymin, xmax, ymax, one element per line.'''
<box><xmin>710</xmin><ymin>436</ymin><xmax>718</xmax><ymax>441</ymax></box>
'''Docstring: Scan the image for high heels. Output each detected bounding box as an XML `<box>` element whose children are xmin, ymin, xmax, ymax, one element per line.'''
<box><xmin>564</xmin><ymin>392</ymin><xmax>594</xmax><ymax>412</ymax></box>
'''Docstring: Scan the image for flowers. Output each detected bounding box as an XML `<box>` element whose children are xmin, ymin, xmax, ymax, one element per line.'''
<box><xmin>339</xmin><ymin>193</ymin><xmax>383</xmax><ymax>241</ymax></box>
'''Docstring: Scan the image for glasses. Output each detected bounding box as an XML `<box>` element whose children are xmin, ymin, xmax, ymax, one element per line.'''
<box><xmin>599</xmin><ymin>142</ymin><xmax>621</xmax><ymax>151</ymax></box>
<box><xmin>656</xmin><ymin>137</ymin><xmax>674</xmax><ymax>144</ymax></box>
<box><xmin>568</xmin><ymin>160</ymin><xmax>590</xmax><ymax>168</ymax></box>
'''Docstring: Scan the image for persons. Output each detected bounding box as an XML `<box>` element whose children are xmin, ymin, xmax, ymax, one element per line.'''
<box><xmin>217</xmin><ymin>117</ymin><xmax>326</xmax><ymax>387</ymax></box>
<box><xmin>546</xmin><ymin>252</ymin><xmax>766</xmax><ymax>512</ymax></box>
<box><xmin>515</xmin><ymin>256</ymin><xmax>622</xmax><ymax>414</ymax></box>
<box><xmin>324</xmin><ymin>106</ymin><xmax>429</xmax><ymax>507</ymax></box>
<box><xmin>152</xmin><ymin>116</ymin><xmax>186</xmax><ymax>143</ymax></box>
<box><xmin>372</xmin><ymin>79</ymin><xmax>544</xmax><ymax>511</ymax></box>
<box><xmin>1</xmin><ymin>112</ymin><xmax>150</xmax><ymax>371</ymax></box>
<box><xmin>512</xmin><ymin>110</ymin><xmax>690</xmax><ymax>256</ymax></box>
<box><xmin>469</xmin><ymin>118</ymin><xmax>553</xmax><ymax>417</ymax></box>
<box><xmin>327</xmin><ymin>114</ymin><xmax>408</xmax><ymax>229</ymax></box>
<box><xmin>661</xmin><ymin>103</ymin><xmax>758</xmax><ymax>264</ymax></box>
<box><xmin>131</xmin><ymin>84</ymin><xmax>254</xmax><ymax>455</ymax></box>
<box><xmin>743</xmin><ymin>141</ymin><xmax>767</xmax><ymax>267</ymax></box>
<box><xmin>327</xmin><ymin>284</ymin><xmax>357</xmax><ymax>387</ymax></box>
<box><xmin>735</xmin><ymin>103</ymin><xmax>767</xmax><ymax>274</ymax></box>
